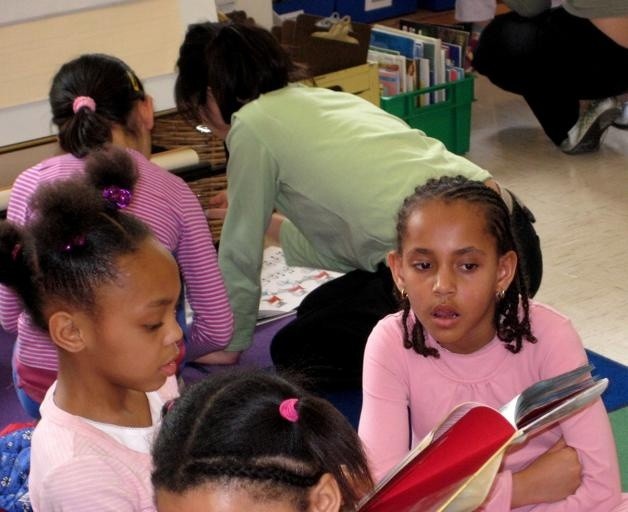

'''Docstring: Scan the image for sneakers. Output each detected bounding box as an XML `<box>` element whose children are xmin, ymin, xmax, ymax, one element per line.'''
<box><xmin>560</xmin><ymin>95</ymin><xmax>627</xmax><ymax>154</ymax></box>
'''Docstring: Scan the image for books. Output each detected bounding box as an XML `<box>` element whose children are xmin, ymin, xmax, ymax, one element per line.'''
<box><xmin>355</xmin><ymin>360</ymin><xmax>609</xmax><ymax>512</ymax></box>
<box><xmin>365</xmin><ymin>19</ymin><xmax>473</xmax><ymax>110</ymax></box>
<box><xmin>183</xmin><ymin>243</ymin><xmax>347</xmax><ymax>333</ymax></box>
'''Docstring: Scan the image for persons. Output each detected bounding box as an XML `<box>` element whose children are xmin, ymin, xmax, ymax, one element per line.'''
<box><xmin>148</xmin><ymin>366</ymin><xmax>371</xmax><ymax>512</ymax></box>
<box><xmin>0</xmin><ymin>53</ymin><xmax>236</xmax><ymax>425</ymax></box>
<box><xmin>172</xmin><ymin>17</ymin><xmax>546</xmax><ymax>398</ymax></box>
<box><xmin>1</xmin><ymin>146</ymin><xmax>190</xmax><ymax>512</ymax></box>
<box><xmin>355</xmin><ymin>172</ymin><xmax>628</xmax><ymax>512</ymax></box>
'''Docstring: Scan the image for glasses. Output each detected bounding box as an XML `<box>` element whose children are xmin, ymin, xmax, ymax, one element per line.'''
<box><xmin>194</xmin><ymin>89</ymin><xmax>212</xmax><ymax>134</ymax></box>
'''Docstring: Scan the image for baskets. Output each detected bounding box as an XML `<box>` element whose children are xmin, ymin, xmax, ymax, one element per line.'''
<box><xmin>149</xmin><ymin>110</ymin><xmax>230</xmax><ymax>245</ymax></box>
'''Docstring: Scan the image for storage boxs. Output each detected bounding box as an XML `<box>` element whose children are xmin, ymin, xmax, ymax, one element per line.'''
<box><xmin>381</xmin><ymin>69</ymin><xmax>477</xmax><ymax>153</ymax></box>
